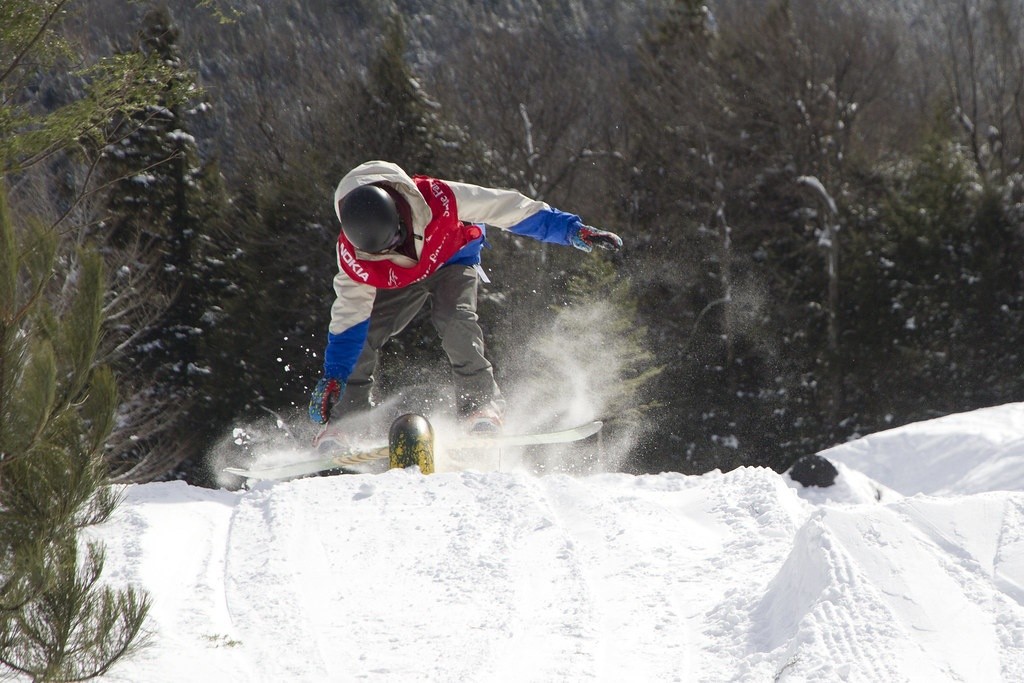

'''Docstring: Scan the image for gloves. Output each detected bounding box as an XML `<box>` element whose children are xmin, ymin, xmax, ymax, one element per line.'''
<box><xmin>309</xmin><ymin>376</ymin><xmax>341</xmax><ymax>424</ymax></box>
<box><xmin>567</xmin><ymin>220</ymin><xmax>623</xmax><ymax>253</ymax></box>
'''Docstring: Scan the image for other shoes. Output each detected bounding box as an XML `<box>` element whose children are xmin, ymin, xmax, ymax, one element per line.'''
<box><xmin>464</xmin><ymin>405</ymin><xmax>503</xmax><ymax>435</ymax></box>
<box><xmin>313</xmin><ymin>427</ymin><xmax>347</xmax><ymax>456</ymax></box>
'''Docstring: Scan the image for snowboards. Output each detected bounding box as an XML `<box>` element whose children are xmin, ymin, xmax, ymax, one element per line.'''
<box><xmin>387</xmin><ymin>413</ymin><xmax>435</xmax><ymax>475</ymax></box>
<box><xmin>222</xmin><ymin>419</ymin><xmax>605</xmax><ymax>482</ymax></box>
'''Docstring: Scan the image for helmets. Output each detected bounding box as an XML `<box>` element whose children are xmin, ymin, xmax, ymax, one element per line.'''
<box><xmin>340</xmin><ymin>185</ymin><xmax>399</xmax><ymax>251</ymax></box>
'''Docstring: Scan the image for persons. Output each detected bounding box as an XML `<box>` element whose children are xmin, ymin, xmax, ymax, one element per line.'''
<box><xmin>308</xmin><ymin>160</ymin><xmax>622</xmax><ymax>477</ymax></box>
